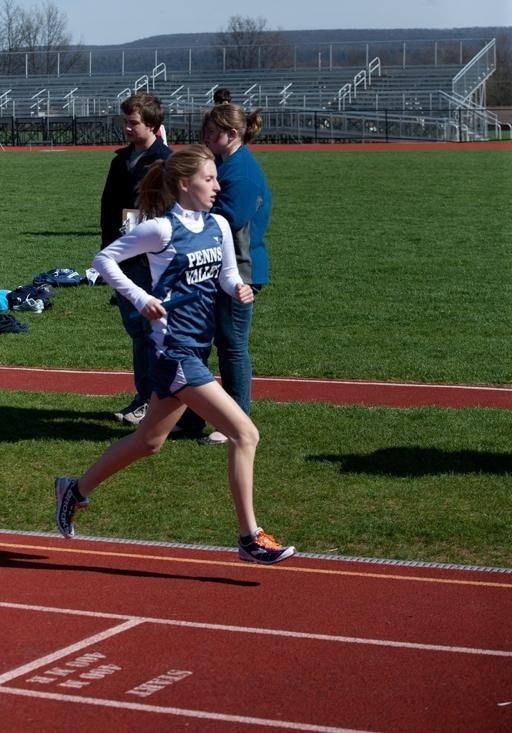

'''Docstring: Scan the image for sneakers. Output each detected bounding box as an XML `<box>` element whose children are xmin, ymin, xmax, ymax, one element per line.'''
<box><xmin>55</xmin><ymin>473</ymin><xmax>91</xmax><ymax>541</ymax></box>
<box><xmin>114</xmin><ymin>390</ymin><xmax>151</xmax><ymax>427</ymax></box>
<box><xmin>237</xmin><ymin>524</ymin><xmax>298</xmax><ymax>564</ymax></box>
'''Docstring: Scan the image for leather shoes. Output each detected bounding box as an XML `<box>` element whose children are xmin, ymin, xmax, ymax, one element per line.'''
<box><xmin>197</xmin><ymin>434</ymin><xmax>230</xmax><ymax>447</ymax></box>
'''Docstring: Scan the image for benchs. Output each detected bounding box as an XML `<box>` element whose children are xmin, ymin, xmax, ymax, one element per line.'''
<box><xmin>0</xmin><ymin>64</ymin><xmax>493</xmax><ymax>118</ymax></box>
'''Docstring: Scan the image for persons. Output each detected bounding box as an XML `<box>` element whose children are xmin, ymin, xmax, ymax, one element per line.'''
<box><xmin>95</xmin><ymin>90</ymin><xmax>175</xmax><ymax>426</ymax></box>
<box><xmin>169</xmin><ymin>101</ymin><xmax>274</xmax><ymax>451</ymax></box>
<box><xmin>52</xmin><ymin>140</ymin><xmax>300</xmax><ymax>567</ymax></box>
<box><xmin>211</xmin><ymin>88</ymin><xmax>233</xmax><ymax>107</ymax></box>
